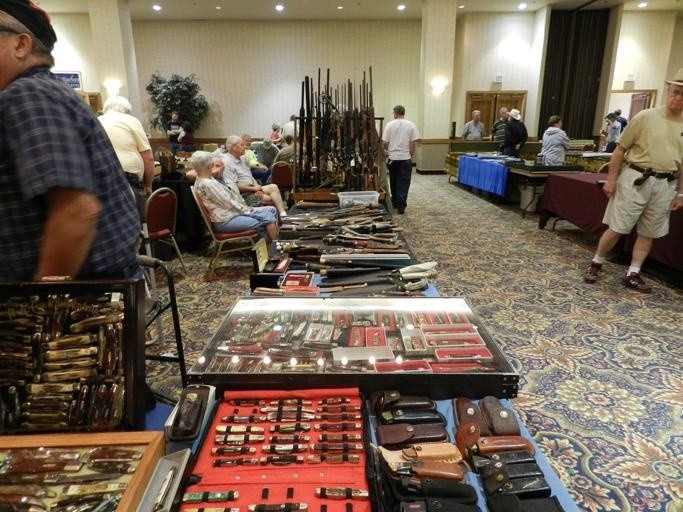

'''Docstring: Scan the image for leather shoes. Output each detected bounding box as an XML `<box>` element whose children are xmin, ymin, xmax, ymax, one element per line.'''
<box><xmin>584</xmin><ymin>261</ymin><xmax>602</xmax><ymax>283</ymax></box>
<box><xmin>622</xmin><ymin>272</ymin><xmax>651</xmax><ymax>293</ymax></box>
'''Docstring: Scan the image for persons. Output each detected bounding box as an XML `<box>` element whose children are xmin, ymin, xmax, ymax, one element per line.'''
<box><xmin>460</xmin><ymin>109</ymin><xmax>487</xmax><ymax>141</ymax></box>
<box><xmin>599</xmin><ymin>112</ymin><xmax>621</xmax><ymax>153</ymax></box>
<box><xmin>489</xmin><ymin>104</ymin><xmax>509</xmax><ymax>141</ymax></box>
<box><xmin>164</xmin><ymin>110</ymin><xmax>307</xmax><ymax>252</ymax></box>
<box><xmin>381</xmin><ymin>105</ymin><xmax>420</xmax><ymax>214</ymax></box>
<box><xmin>96</xmin><ymin>94</ymin><xmax>155</xmax><ymax>280</ymax></box>
<box><xmin>501</xmin><ymin>108</ymin><xmax>529</xmax><ymax>157</ymax></box>
<box><xmin>540</xmin><ymin>115</ymin><xmax>571</xmax><ymax>166</ymax></box>
<box><xmin>583</xmin><ymin>66</ymin><xmax>683</xmax><ymax>294</ymax></box>
<box><xmin>0</xmin><ymin>0</ymin><xmax>142</xmax><ymax>281</ymax></box>
<box><xmin>614</xmin><ymin>109</ymin><xmax>628</xmax><ymax>135</ymax></box>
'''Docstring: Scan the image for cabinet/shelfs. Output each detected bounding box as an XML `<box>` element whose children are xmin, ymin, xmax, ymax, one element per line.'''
<box><xmin>70</xmin><ymin>89</ymin><xmax>103</xmax><ymax>117</ymax></box>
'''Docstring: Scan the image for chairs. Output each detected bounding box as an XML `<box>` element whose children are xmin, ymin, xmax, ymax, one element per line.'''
<box><xmin>271</xmin><ymin>161</ymin><xmax>293</xmax><ymax>206</ymax></box>
<box><xmin>138</xmin><ymin>184</ymin><xmax>186</xmax><ymax>285</ymax></box>
<box><xmin>133</xmin><ymin>256</ymin><xmax>194</xmax><ymax>431</ymax></box>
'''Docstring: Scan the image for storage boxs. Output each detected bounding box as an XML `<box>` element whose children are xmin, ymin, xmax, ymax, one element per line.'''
<box><xmin>0</xmin><ymin>275</ymin><xmax>149</xmax><ymax>431</ymax></box>
<box><xmin>0</xmin><ymin>431</ymin><xmax>164</xmax><ymax>512</ymax></box>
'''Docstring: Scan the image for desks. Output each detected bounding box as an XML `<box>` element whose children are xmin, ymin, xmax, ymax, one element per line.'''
<box><xmin>171</xmin><ymin>187</ymin><xmax>586</xmax><ymax>510</ymax></box>
<box><xmin>441</xmin><ymin>145</ymin><xmax>683</xmax><ymax>283</ymax></box>
<box><xmin>142</xmin><ymin>171</ymin><xmax>210</xmax><ymax>259</ymax></box>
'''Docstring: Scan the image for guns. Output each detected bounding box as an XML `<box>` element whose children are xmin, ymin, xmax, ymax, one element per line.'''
<box><xmin>297</xmin><ymin>66</ymin><xmax>379</xmax><ymax>190</ymax></box>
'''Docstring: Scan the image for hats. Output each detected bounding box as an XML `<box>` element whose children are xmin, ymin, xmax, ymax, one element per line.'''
<box><xmin>263</xmin><ymin>139</ymin><xmax>272</xmax><ymax>150</ymax></box>
<box><xmin>1</xmin><ymin>1</ymin><xmax>57</xmax><ymax>48</ymax></box>
<box><xmin>506</xmin><ymin>109</ymin><xmax>521</xmax><ymax>121</ymax></box>
<box><xmin>663</xmin><ymin>68</ymin><xmax>683</xmax><ymax>88</ymax></box>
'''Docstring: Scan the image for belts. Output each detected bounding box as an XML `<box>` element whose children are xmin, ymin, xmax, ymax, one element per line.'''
<box><xmin>630</xmin><ymin>164</ymin><xmax>676</xmax><ymax>180</ymax></box>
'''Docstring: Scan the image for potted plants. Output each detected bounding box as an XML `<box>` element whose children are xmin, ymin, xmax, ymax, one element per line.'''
<box><xmin>145</xmin><ymin>69</ymin><xmax>210</xmax><ymax>148</ymax></box>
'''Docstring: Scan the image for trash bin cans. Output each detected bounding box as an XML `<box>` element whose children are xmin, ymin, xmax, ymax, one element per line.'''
<box><xmin>519</xmin><ymin>183</ymin><xmax>545</xmax><ymax>212</ymax></box>
